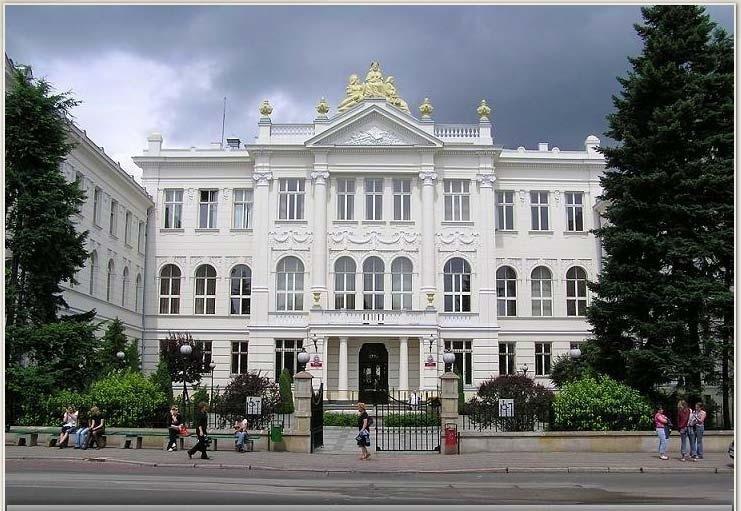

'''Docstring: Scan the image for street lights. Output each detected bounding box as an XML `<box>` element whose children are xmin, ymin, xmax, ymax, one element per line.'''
<box><xmin>116</xmin><ymin>349</ymin><xmax>125</xmax><ymax>368</ymax></box>
<box><xmin>209</xmin><ymin>361</ymin><xmax>216</xmax><ymax>402</ymax></box>
<box><xmin>522</xmin><ymin>364</ymin><xmax>529</xmax><ymax>396</ymax></box>
<box><xmin>180</xmin><ymin>342</ymin><xmax>193</xmax><ymax>404</ymax></box>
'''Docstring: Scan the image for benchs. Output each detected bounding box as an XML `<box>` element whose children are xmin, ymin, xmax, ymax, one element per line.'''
<box><xmin>9</xmin><ymin>427</ymin><xmax>260</xmax><ymax>453</ymax></box>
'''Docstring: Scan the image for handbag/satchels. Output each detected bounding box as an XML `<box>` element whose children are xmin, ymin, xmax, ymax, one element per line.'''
<box><xmin>179</xmin><ymin>424</ymin><xmax>189</xmax><ymax>436</ymax></box>
<box><xmin>366</xmin><ymin>413</ymin><xmax>373</xmax><ymax>427</ymax></box>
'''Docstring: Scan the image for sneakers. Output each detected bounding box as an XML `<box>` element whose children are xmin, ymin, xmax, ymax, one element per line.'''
<box><xmin>55</xmin><ymin>442</ymin><xmax>64</xmax><ymax>448</ymax></box>
<box><xmin>167</xmin><ymin>442</ymin><xmax>176</xmax><ymax>452</ymax></box>
<box><xmin>359</xmin><ymin>453</ymin><xmax>371</xmax><ymax>461</ymax></box>
<box><xmin>200</xmin><ymin>454</ymin><xmax>209</xmax><ymax>459</ymax></box>
<box><xmin>186</xmin><ymin>450</ymin><xmax>192</xmax><ymax>459</ymax></box>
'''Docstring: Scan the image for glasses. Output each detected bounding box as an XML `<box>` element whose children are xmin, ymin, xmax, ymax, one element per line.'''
<box><xmin>175</xmin><ymin>409</ymin><xmax>179</xmax><ymax>411</ymax></box>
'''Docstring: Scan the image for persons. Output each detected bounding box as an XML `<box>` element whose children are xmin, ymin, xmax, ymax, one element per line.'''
<box><xmin>55</xmin><ymin>405</ymin><xmax>78</xmax><ymax>449</ymax></box>
<box><xmin>356</xmin><ymin>402</ymin><xmax>371</xmax><ymax>460</ymax></box>
<box><xmin>677</xmin><ymin>400</ymin><xmax>697</xmax><ymax>462</ymax></box>
<box><xmin>384</xmin><ymin>75</ymin><xmax>410</xmax><ymax>114</ymax></box>
<box><xmin>654</xmin><ymin>404</ymin><xmax>669</xmax><ymax>460</ymax></box>
<box><xmin>167</xmin><ymin>405</ymin><xmax>185</xmax><ymax>452</ymax></box>
<box><xmin>234</xmin><ymin>414</ymin><xmax>247</xmax><ymax>452</ymax></box>
<box><xmin>693</xmin><ymin>402</ymin><xmax>706</xmax><ymax>459</ymax></box>
<box><xmin>74</xmin><ymin>408</ymin><xmax>91</xmax><ymax>450</ymax></box>
<box><xmin>336</xmin><ymin>74</ymin><xmax>365</xmax><ymax>109</ymax></box>
<box><xmin>187</xmin><ymin>402</ymin><xmax>211</xmax><ymax>459</ymax></box>
<box><xmin>87</xmin><ymin>407</ymin><xmax>105</xmax><ymax>450</ymax></box>
<box><xmin>364</xmin><ymin>61</ymin><xmax>384</xmax><ymax>98</ymax></box>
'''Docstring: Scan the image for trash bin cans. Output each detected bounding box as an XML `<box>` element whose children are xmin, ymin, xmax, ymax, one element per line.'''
<box><xmin>444</xmin><ymin>423</ymin><xmax>457</xmax><ymax>455</ymax></box>
<box><xmin>271</xmin><ymin>420</ymin><xmax>282</xmax><ymax>442</ymax></box>
